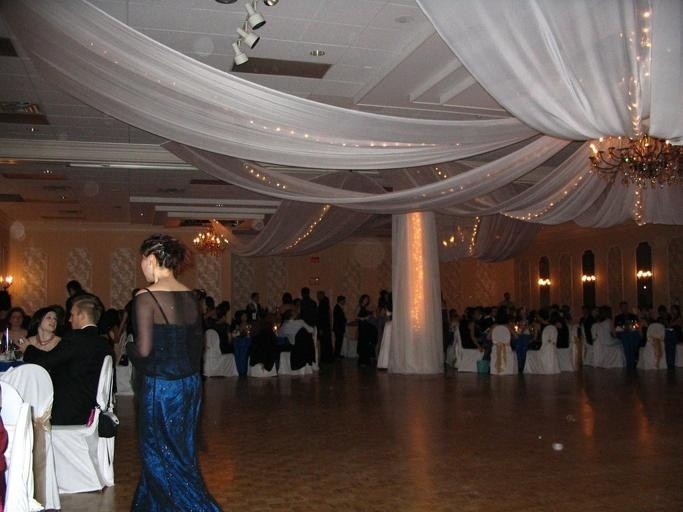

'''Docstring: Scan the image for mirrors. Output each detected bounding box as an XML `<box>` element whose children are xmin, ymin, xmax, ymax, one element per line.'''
<box><xmin>477</xmin><ymin>334</ymin><xmax>534</xmax><ymax>372</ymax></box>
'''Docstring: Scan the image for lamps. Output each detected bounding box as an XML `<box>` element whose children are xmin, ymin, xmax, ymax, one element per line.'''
<box><xmin>0</xmin><ymin>276</ymin><xmax>13</xmax><ymax>291</ymax></box>
<box><xmin>588</xmin><ymin>133</ymin><xmax>683</xmax><ymax>190</ymax></box>
<box><xmin>582</xmin><ymin>275</ymin><xmax>596</xmax><ymax>282</ymax></box>
<box><xmin>636</xmin><ymin>270</ymin><xmax>652</xmax><ymax>290</ymax></box>
<box><xmin>236</xmin><ymin>27</ymin><xmax>260</xmax><ymax>49</ymax></box>
<box><xmin>244</xmin><ymin>3</ymin><xmax>265</xmax><ymax>30</ymax></box>
<box><xmin>229</xmin><ymin>42</ymin><xmax>249</xmax><ymax>65</ymax></box>
<box><xmin>538</xmin><ymin>278</ymin><xmax>550</xmax><ymax>285</ymax></box>
<box><xmin>193</xmin><ymin>227</ymin><xmax>228</xmax><ymax>257</ymax></box>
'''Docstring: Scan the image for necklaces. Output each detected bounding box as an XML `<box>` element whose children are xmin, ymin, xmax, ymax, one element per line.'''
<box><xmin>37</xmin><ymin>332</ymin><xmax>55</xmax><ymax>345</ymax></box>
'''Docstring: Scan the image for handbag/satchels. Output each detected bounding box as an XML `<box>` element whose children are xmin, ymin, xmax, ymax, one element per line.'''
<box><xmin>83</xmin><ymin>375</ymin><xmax>120</xmax><ymax>438</ymax></box>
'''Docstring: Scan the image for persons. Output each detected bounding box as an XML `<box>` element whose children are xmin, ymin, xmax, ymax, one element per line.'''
<box><xmin>247</xmin><ymin>293</ymin><xmax>261</xmax><ymax>313</ymax></box>
<box><xmin>656</xmin><ymin>304</ymin><xmax>683</xmax><ymax>341</ymax></box>
<box><xmin>107</xmin><ymin>286</ymin><xmax>139</xmax><ymax>365</ymax></box>
<box><xmin>277</xmin><ymin>309</ymin><xmax>314</xmax><ymax>346</ymax></box>
<box><xmin>354</xmin><ymin>295</ymin><xmax>369</xmax><ymax>320</ymax></box>
<box><xmin>316</xmin><ymin>290</ymin><xmax>331</xmax><ymax>346</ymax></box>
<box><xmin>65</xmin><ymin>282</ymin><xmax>86</xmax><ymax>303</ymax></box>
<box><xmin>595</xmin><ymin>305</ymin><xmax>622</xmax><ymax>345</ymax></box>
<box><xmin>450</xmin><ymin>306</ymin><xmax>498</xmax><ymax>360</ymax></box>
<box><xmin>614</xmin><ymin>302</ymin><xmax>637</xmax><ymax>326</ymax></box>
<box><xmin>0</xmin><ymin>385</ymin><xmax>9</xmax><ymax>511</ymax></box>
<box><xmin>127</xmin><ymin>233</ymin><xmax>221</xmax><ymax>512</ymax></box>
<box><xmin>498</xmin><ymin>292</ymin><xmax>527</xmax><ymax>324</ymax></box>
<box><xmin>0</xmin><ymin>308</ymin><xmax>28</xmax><ymax>349</ymax></box>
<box><xmin>24</xmin><ymin>308</ymin><xmax>62</xmax><ymax>353</ymax></box>
<box><xmin>580</xmin><ymin>306</ymin><xmax>594</xmax><ymax>334</ymax></box>
<box><xmin>379</xmin><ymin>289</ymin><xmax>392</xmax><ymax>318</ymax></box>
<box><xmin>19</xmin><ymin>293</ymin><xmax>110</xmax><ymax>426</ymax></box>
<box><xmin>528</xmin><ymin>304</ymin><xmax>571</xmax><ymax>349</ymax></box>
<box><xmin>299</xmin><ymin>287</ymin><xmax>316</xmax><ymax>325</ymax></box>
<box><xmin>333</xmin><ymin>295</ymin><xmax>346</xmax><ymax>358</ymax></box>
<box><xmin>276</xmin><ymin>292</ymin><xmax>298</xmax><ymax>319</ymax></box>
<box><xmin>205</xmin><ymin>296</ymin><xmax>249</xmax><ymax>352</ymax></box>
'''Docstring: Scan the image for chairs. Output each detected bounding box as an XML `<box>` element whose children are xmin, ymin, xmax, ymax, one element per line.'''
<box><xmin>341</xmin><ymin>336</ymin><xmax>357</xmax><ymax>358</ymax></box>
<box><xmin>50</xmin><ymin>355</ymin><xmax>114</xmax><ymax>494</ymax></box>
<box><xmin>4</xmin><ymin>403</ymin><xmax>45</xmax><ymax>512</ymax></box>
<box><xmin>591</xmin><ymin>324</ymin><xmax>626</xmax><ymax>368</ymax></box>
<box><xmin>636</xmin><ymin>324</ymin><xmax>668</xmax><ymax>370</ymax></box>
<box><xmin>247</xmin><ymin>323</ymin><xmax>277</xmax><ymax>377</ymax></box>
<box><xmin>453</xmin><ymin>325</ymin><xmax>484</xmax><ymax>372</ymax></box>
<box><xmin>312</xmin><ymin>327</ymin><xmax>319</xmax><ymax>371</ymax></box>
<box><xmin>490</xmin><ymin>326</ymin><xmax>518</xmax><ymax>375</ymax></box>
<box><xmin>203</xmin><ymin>329</ymin><xmax>239</xmax><ymax>378</ymax></box>
<box><xmin>0</xmin><ymin>382</ymin><xmax>23</xmax><ymax>485</ymax></box>
<box><xmin>278</xmin><ymin>328</ymin><xmax>313</xmax><ymax>375</ymax></box>
<box><xmin>557</xmin><ymin>325</ymin><xmax>581</xmax><ymax>372</ymax></box>
<box><xmin>114</xmin><ymin>332</ymin><xmax>127</xmax><ymax>354</ymax></box>
<box><xmin>115</xmin><ymin>335</ymin><xmax>134</xmax><ymax>395</ymax></box>
<box><xmin>523</xmin><ymin>325</ymin><xmax>560</xmax><ymax>375</ymax></box>
<box><xmin>581</xmin><ymin>324</ymin><xmax>593</xmax><ymax>366</ymax></box>
